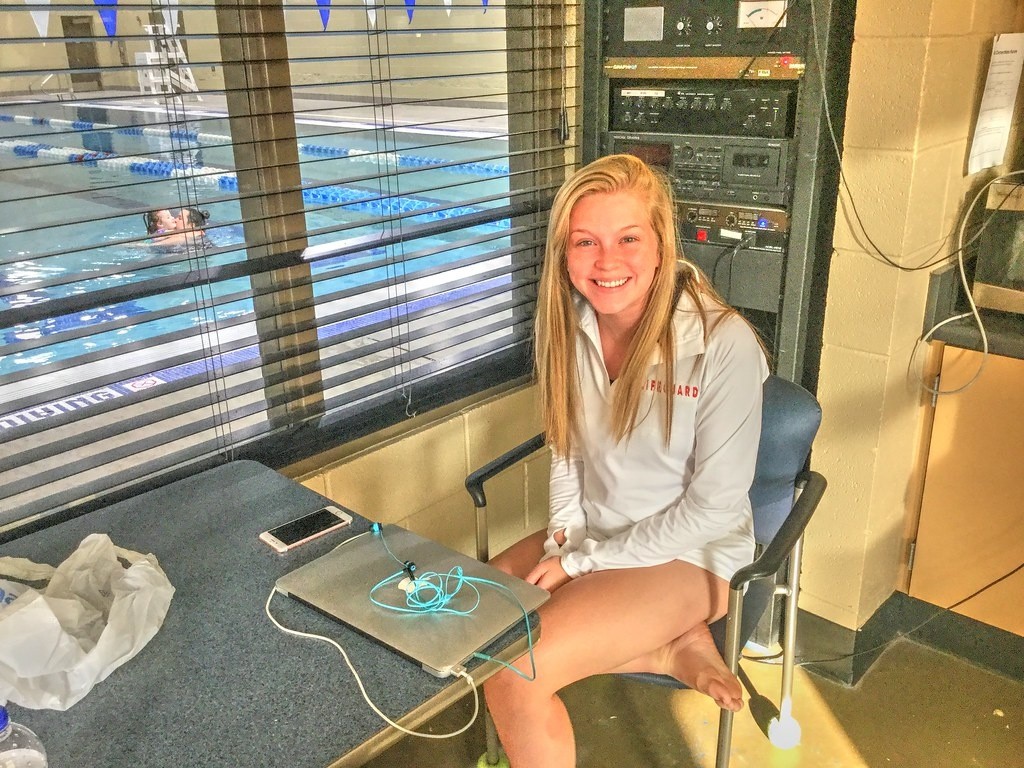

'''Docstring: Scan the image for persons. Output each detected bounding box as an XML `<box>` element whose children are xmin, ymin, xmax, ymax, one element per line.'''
<box><xmin>478</xmin><ymin>151</ymin><xmax>770</xmax><ymax>767</ymax></box>
<box><xmin>144</xmin><ymin>206</ymin><xmax>211</xmax><ymax>247</ymax></box>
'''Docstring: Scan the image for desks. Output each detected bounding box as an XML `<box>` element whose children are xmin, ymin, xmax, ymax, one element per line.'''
<box><xmin>1</xmin><ymin>459</ymin><xmax>542</xmax><ymax>768</ymax></box>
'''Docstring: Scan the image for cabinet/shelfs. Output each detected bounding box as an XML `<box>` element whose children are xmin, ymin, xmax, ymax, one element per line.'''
<box><xmin>909</xmin><ymin>345</ymin><xmax>1024</xmax><ymax>638</ymax></box>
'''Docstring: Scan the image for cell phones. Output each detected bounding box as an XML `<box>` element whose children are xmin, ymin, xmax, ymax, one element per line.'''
<box><xmin>258</xmin><ymin>505</ymin><xmax>353</xmax><ymax>554</ymax></box>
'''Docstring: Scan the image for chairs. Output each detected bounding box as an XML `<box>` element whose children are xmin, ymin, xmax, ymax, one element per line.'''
<box><xmin>464</xmin><ymin>376</ymin><xmax>827</xmax><ymax>768</ymax></box>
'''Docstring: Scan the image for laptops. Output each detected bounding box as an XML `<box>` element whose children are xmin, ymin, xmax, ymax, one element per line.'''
<box><xmin>276</xmin><ymin>523</ymin><xmax>551</xmax><ymax>679</ymax></box>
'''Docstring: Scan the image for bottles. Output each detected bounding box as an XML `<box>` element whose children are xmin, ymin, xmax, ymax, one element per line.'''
<box><xmin>0</xmin><ymin>707</ymin><xmax>48</xmax><ymax>768</ymax></box>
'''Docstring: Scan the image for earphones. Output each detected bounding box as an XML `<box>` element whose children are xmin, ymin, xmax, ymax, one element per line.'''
<box><xmin>370</xmin><ymin>522</ymin><xmax>382</xmax><ymax>532</ymax></box>
<box><xmin>405</xmin><ymin>561</ymin><xmax>415</xmax><ymax>571</ymax></box>
